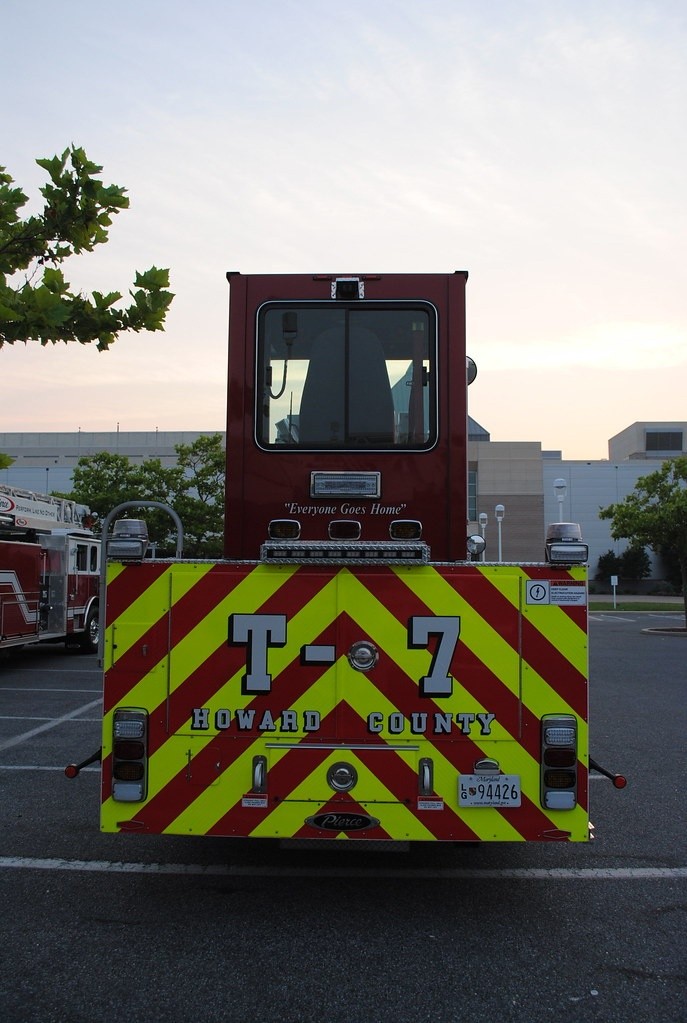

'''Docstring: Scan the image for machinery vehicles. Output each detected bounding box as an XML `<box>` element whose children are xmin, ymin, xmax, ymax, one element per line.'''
<box><xmin>63</xmin><ymin>270</ymin><xmax>627</xmax><ymax>844</ymax></box>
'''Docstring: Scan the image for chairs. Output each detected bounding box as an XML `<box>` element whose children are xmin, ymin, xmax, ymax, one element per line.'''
<box><xmin>298</xmin><ymin>340</ymin><xmax>396</xmax><ymax>450</ymax></box>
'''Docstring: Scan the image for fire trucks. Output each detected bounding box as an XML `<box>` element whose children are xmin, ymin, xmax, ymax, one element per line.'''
<box><xmin>0</xmin><ymin>484</ymin><xmax>114</xmax><ymax>655</ymax></box>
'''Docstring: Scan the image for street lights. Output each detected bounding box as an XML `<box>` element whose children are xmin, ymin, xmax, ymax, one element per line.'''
<box><xmin>552</xmin><ymin>478</ymin><xmax>568</xmax><ymax>523</ymax></box>
<box><xmin>478</xmin><ymin>512</ymin><xmax>489</xmax><ymax>561</ymax></box>
<box><xmin>494</xmin><ymin>504</ymin><xmax>505</xmax><ymax>562</ymax></box>
